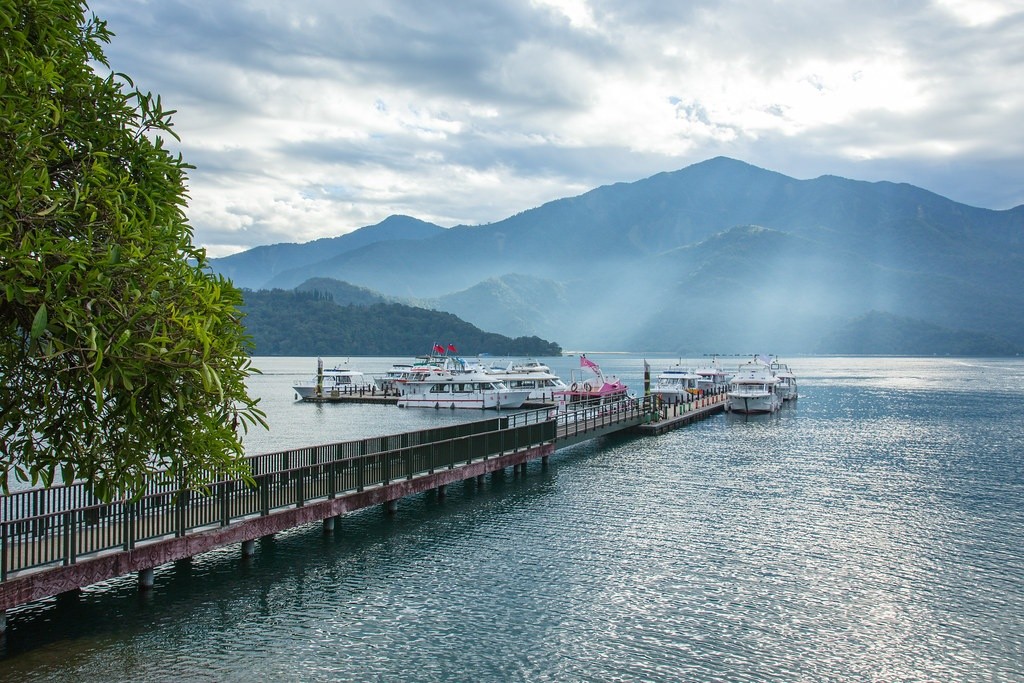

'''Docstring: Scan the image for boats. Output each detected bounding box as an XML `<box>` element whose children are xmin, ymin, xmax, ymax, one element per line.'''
<box><xmin>650</xmin><ymin>354</ymin><xmax>799</xmax><ymax>413</ymax></box>
<box><xmin>372</xmin><ymin>341</ymin><xmax>566</xmax><ymax>410</ymax></box>
<box><xmin>292</xmin><ymin>355</ymin><xmax>371</xmax><ymax>400</ymax></box>
<box><xmin>551</xmin><ymin>354</ymin><xmax>628</xmax><ymax>406</ymax></box>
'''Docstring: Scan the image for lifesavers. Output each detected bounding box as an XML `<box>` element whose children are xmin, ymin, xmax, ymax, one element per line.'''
<box><xmin>571</xmin><ymin>383</ymin><xmax>578</xmax><ymax>392</ymax></box>
<box><xmin>584</xmin><ymin>383</ymin><xmax>592</xmax><ymax>391</ymax></box>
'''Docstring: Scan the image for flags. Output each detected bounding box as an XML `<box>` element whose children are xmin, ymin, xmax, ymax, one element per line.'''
<box><xmin>447</xmin><ymin>343</ymin><xmax>457</xmax><ymax>352</ymax></box>
<box><xmin>433</xmin><ymin>343</ymin><xmax>445</xmax><ymax>355</ymax></box>
